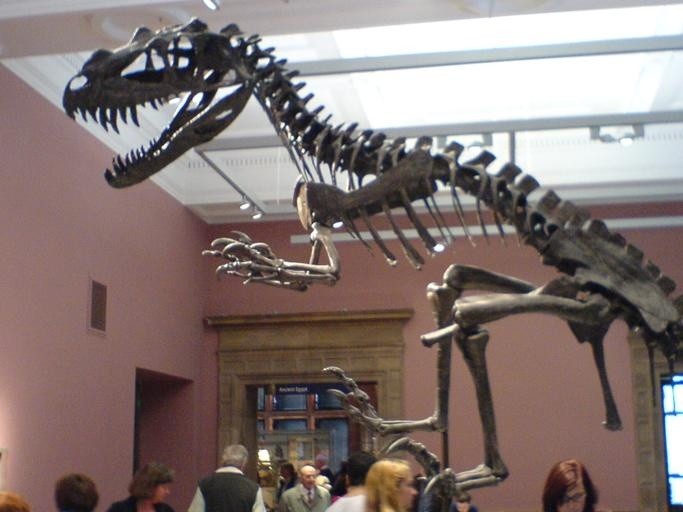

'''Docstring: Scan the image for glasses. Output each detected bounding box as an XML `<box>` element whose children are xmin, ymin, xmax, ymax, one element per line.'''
<box><xmin>562</xmin><ymin>493</ymin><xmax>586</xmax><ymax>502</ymax></box>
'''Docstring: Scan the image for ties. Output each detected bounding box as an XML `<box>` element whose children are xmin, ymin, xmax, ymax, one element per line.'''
<box><xmin>307</xmin><ymin>491</ymin><xmax>313</xmax><ymax>505</ymax></box>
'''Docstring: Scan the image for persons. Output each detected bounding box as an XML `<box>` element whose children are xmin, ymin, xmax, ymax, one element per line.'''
<box><xmin>278</xmin><ymin>465</ymin><xmax>331</xmax><ymax>512</ymax></box>
<box><xmin>450</xmin><ymin>491</ymin><xmax>478</xmax><ymax>512</ymax></box>
<box><xmin>277</xmin><ymin>462</ymin><xmax>297</xmax><ymax>503</ymax></box>
<box><xmin>257</xmin><ymin>470</ymin><xmax>279</xmax><ymax>512</ymax></box>
<box><xmin>1</xmin><ymin>492</ymin><xmax>32</xmax><ymax>512</ymax></box>
<box><xmin>314</xmin><ymin>455</ymin><xmax>333</xmax><ymax>486</ymax></box>
<box><xmin>186</xmin><ymin>445</ymin><xmax>265</xmax><ymax>512</ymax></box>
<box><xmin>326</xmin><ymin>450</ymin><xmax>376</xmax><ymax>512</ymax></box>
<box><xmin>54</xmin><ymin>475</ymin><xmax>98</xmax><ymax>512</ymax></box>
<box><xmin>542</xmin><ymin>461</ymin><xmax>598</xmax><ymax>512</ymax></box>
<box><xmin>365</xmin><ymin>457</ymin><xmax>418</xmax><ymax>511</ymax></box>
<box><xmin>328</xmin><ymin>469</ymin><xmax>350</xmax><ymax>502</ymax></box>
<box><xmin>316</xmin><ymin>475</ymin><xmax>331</xmax><ymax>493</ymax></box>
<box><xmin>104</xmin><ymin>461</ymin><xmax>178</xmax><ymax>512</ymax></box>
